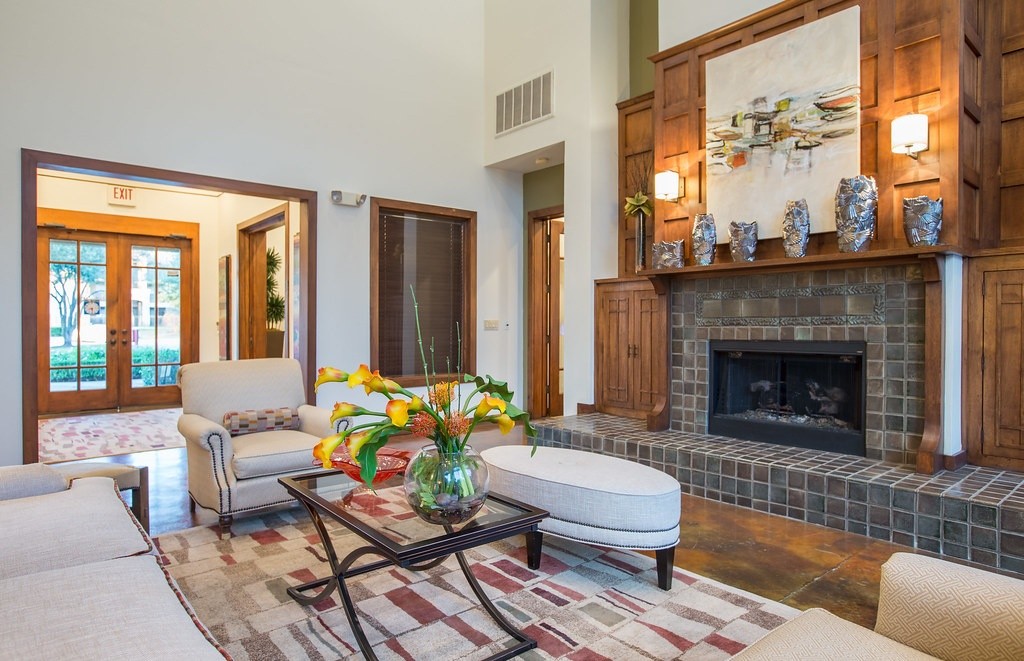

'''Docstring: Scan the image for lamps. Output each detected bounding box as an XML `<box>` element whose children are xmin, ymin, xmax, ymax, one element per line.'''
<box><xmin>330</xmin><ymin>190</ymin><xmax>366</xmax><ymax>207</ymax></box>
<box><xmin>655</xmin><ymin>168</ymin><xmax>685</xmax><ymax>203</ymax></box>
<box><xmin>890</xmin><ymin>113</ymin><xmax>929</xmax><ymax>158</ymax></box>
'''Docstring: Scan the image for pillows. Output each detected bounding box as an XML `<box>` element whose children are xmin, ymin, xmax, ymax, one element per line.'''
<box><xmin>223</xmin><ymin>407</ymin><xmax>300</xmax><ymax>434</ymax></box>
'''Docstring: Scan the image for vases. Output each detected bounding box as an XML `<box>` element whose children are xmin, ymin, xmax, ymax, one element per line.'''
<box><xmin>403</xmin><ymin>443</ymin><xmax>489</xmax><ymax>525</ymax></box>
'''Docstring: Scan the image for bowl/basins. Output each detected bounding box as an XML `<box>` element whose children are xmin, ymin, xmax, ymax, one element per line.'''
<box><xmin>312</xmin><ymin>454</ymin><xmax>410</xmax><ymax>483</ymax></box>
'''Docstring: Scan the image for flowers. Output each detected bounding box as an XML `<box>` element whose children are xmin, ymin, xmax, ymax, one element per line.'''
<box><xmin>313</xmin><ymin>285</ymin><xmax>537</xmax><ymax>495</ymax></box>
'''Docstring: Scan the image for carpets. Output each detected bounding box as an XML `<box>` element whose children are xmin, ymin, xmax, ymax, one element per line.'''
<box><xmin>152</xmin><ymin>479</ymin><xmax>805</xmax><ymax>660</ymax></box>
<box><xmin>38</xmin><ymin>407</ymin><xmax>187</xmax><ymax>463</ymax></box>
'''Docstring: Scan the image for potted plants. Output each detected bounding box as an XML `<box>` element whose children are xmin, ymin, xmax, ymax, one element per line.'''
<box><xmin>267</xmin><ymin>245</ymin><xmax>284</xmax><ymax>358</ymax></box>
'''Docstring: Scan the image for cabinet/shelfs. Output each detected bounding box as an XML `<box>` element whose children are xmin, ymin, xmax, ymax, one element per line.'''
<box><xmin>593</xmin><ymin>276</ymin><xmax>665</xmax><ymax>417</ymax></box>
<box><xmin>962</xmin><ymin>247</ymin><xmax>1024</xmax><ymax>473</ymax></box>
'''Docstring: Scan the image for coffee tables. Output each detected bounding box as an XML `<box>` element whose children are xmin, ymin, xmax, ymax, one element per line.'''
<box><xmin>277</xmin><ymin>465</ymin><xmax>550</xmax><ymax>661</ymax></box>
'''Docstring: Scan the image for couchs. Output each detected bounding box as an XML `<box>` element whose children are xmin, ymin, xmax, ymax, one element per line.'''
<box><xmin>0</xmin><ymin>463</ymin><xmax>234</xmax><ymax>661</ymax></box>
<box><xmin>725</xmin><ymin>551</ymin><xmax>1024</xmax><ymax>661</ymax></box>
<box><xmin>176</xmin><ymin>356</ymin><xmax>353</xmax><ymax>539</ymax></box>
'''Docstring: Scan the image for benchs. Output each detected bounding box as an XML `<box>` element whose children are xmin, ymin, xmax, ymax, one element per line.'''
<box><xmin>479</xmin><ymin>444</ymin><xmax>681</xmax><ymax>590</ymax></box>
